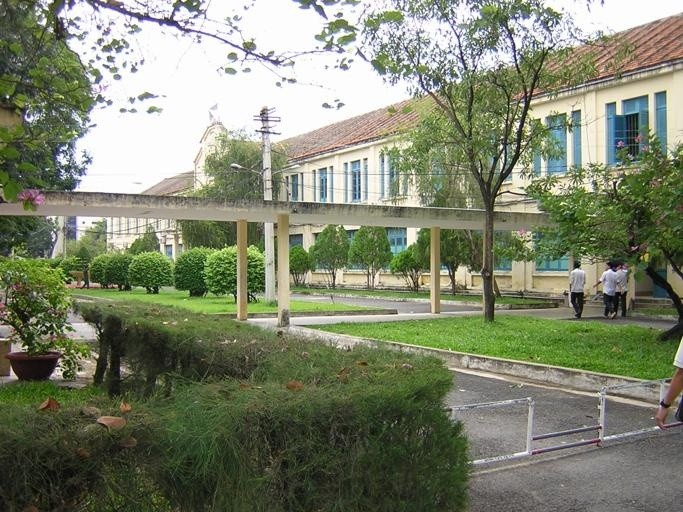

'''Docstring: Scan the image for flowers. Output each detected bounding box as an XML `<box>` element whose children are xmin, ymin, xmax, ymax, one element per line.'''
<box><xmin>0</xmin><ymin>256</ymin><xmax>92</xmax><ymax>380</ymax></box>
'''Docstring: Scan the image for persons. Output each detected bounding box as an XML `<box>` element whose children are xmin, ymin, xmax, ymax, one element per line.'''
<box><xmin>569</xmin><ymin>260</ymin><xmax>585</xmax><ymax>318</ymax></box>
<box><xmin>613</xmin><ymin>262</ymin><xmax>629</xmax><ymax>317</ymax></box>
<box><xmin>653</xmin><ymin>336</ymin><xmax>682</xmax><ymax>430</ymax></box>
<box><xmin>592</xmin><ymin>261</ymin><xmax>619</xmax><ymax>319</ymax></box>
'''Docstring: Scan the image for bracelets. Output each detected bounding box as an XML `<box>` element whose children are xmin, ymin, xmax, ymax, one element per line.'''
<box><xmin>659</xmin><ymin>399</ymin><xmax>672</xmax><ymax>408</ymax></box>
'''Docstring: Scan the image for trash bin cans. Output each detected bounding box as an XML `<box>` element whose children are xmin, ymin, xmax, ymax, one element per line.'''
<box><xmin>562</xmin><ymin>289</ymin><xmax>570</xmax><ymax>306</ymax></box>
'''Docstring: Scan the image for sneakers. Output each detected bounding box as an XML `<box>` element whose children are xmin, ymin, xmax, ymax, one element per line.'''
<box><xmin>573</xmin><ymin>311</ymin><xmax>626</xmax><ymax>320</ymax></box>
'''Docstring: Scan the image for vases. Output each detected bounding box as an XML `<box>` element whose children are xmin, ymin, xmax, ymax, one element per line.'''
<box><xmin>4</xmin><ymin>349</ymin><xmax>60</xmax><ymax>381</ymax></box>
